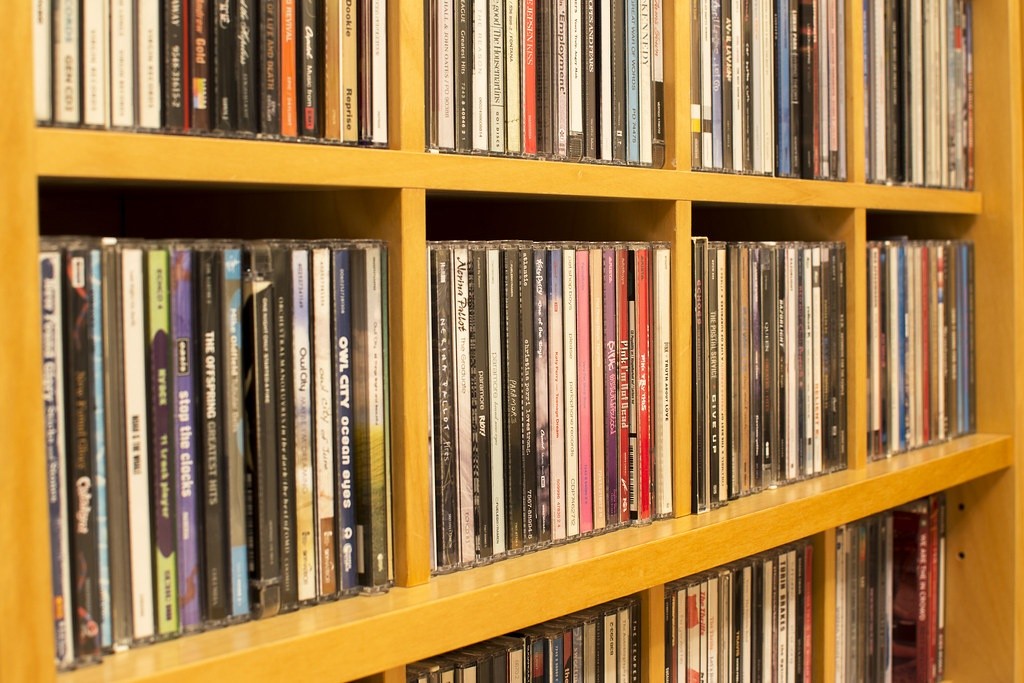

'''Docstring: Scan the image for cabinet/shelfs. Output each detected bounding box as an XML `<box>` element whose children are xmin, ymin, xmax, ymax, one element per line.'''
<box><xmin>0</xmin><ymin>0</ymin><xmax>1024</xmax><ymax>683</ymax></box>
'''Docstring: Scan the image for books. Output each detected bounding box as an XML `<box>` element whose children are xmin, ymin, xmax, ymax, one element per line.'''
<box><xmin>42</xmin><ymin>238</ymin><xmax>975</xmax><ymax>683</ymax></box>
<box><xmin>31</xmin><ymin>0</ymin><xmax>976</xmax><ymax>193</ymax></box>
